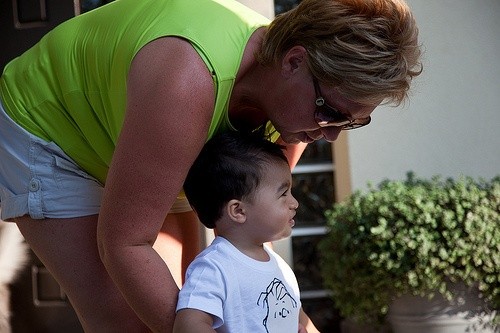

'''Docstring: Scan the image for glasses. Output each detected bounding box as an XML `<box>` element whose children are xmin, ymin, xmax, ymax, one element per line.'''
<box><xmin>313</xmin><ymin>73</ymin><xmax>371</xmax><ymax>131</ymax></box>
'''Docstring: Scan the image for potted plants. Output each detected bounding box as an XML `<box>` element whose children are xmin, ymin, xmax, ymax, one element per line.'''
<box><xmin>316</xmin><ymin>171</ymin><xmax>500</xmax><ymax>333</ymax></box>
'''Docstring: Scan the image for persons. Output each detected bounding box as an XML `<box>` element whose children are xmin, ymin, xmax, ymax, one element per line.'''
<box><xmin>0</xmin><ymin>0</ymin><xmax>424</xmax><ymax>333</ymax></box>
<box><xmin>172</xmin><ymin>132</ymin><xmax>323</xmax><ymax>333</ymax></box>
